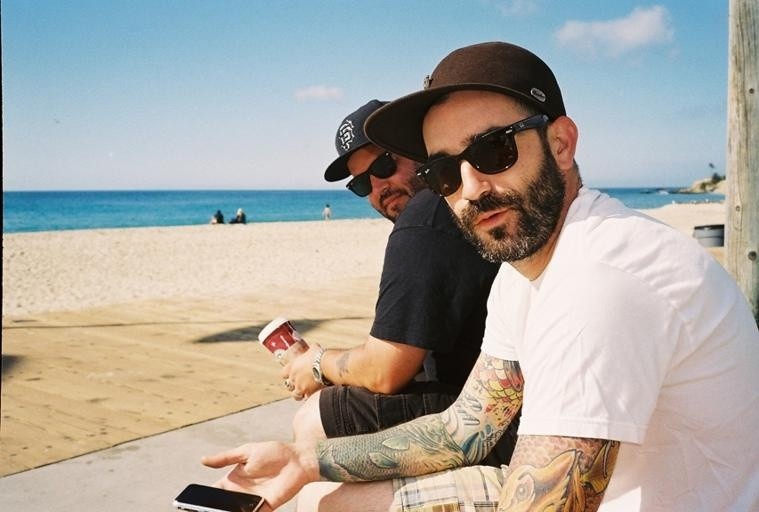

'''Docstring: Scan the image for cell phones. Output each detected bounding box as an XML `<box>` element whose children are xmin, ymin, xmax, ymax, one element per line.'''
<box><xmin>172</xmin><ymin>484</ymin><xmax>265</xmax><ymax>512</ymax></box>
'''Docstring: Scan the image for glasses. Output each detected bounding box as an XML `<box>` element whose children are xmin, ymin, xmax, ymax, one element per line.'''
<box><xmin>345</xmin><ymin>153</ymin><xmax>398</xmax><ymax>197</ymax></box>
<box><xmin>415</xmin><ymin>115</ymin><xmax>549</xmax><ymax>196</ymax></box>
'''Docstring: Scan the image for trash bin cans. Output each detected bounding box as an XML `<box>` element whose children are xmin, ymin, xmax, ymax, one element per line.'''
<box><xmin>692</xmin><ymin>224</ymin><xmax>724</xmax><ymax>248</ymax></box>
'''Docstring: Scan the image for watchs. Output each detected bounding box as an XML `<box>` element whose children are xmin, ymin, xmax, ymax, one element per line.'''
<box><xmin>313</xmin><ymin>348</ymin><xmax>330</xmax><ymax>386</ymax></box>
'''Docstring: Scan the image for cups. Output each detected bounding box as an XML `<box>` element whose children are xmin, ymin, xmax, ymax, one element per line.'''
<box><xmin>257</xmin><ymin>315</ymin><xmax>310</xmax><ymax>367</ymax></box>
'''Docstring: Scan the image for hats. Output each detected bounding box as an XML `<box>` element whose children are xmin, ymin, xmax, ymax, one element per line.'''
<box><xmin>322</xmin><ymin>100</ymin><xmax>389</xmax><ymax>182</ymax></box>
<box><xmin>363</xmin><ymin>42</ymin><xmax>569</xmax><ymax>165</ymax></box>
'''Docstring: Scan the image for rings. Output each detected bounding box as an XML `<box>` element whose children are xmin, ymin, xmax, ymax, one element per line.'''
<box><xmin>284</xmin><ymin>379</ymin><xmax>293</xmax><ymax>390</ymax></box>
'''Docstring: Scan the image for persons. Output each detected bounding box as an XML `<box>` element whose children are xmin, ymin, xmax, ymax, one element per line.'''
<box><xmin>210</xmin><ymin>209</ymin><xmax>246</xmax><ymax>224</ymax></box>
<box><xmin>281</xmin><ymin>100</ymin><xmax>501</xmax><ymax>440</ymax></box>
<box><xmin>201</xmin><ymin>40</ymin><xmax>758</xmax><ymax>510</ymax></box>
<box><xmin>324</xmin><ymin>204</ymin><xmax>331</xmax><ymax>219</ymax></box>
